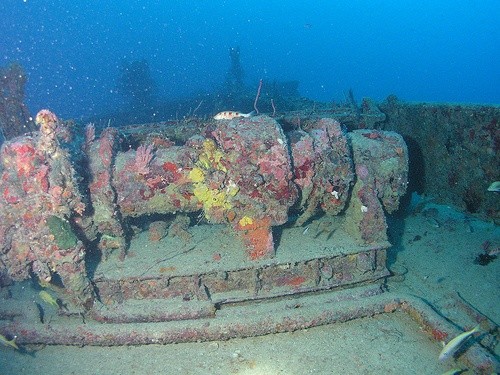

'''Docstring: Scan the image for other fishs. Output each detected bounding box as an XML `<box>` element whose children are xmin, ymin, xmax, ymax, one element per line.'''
<box><xmin>1</xmin><ymin>334</ymin><xmax>20</xmax><ymax>351</ymax></box>
<box><xmin>311</xmin><ymin>216</ymin><xmax>333</xmax><ymax>232</ymax></box>
<box><xmin>211</xmin><ymin>107</ymin><xmax>256</xmax><ymax>121</ymax></box>
<box><xmin>420</xmin><ymin>201</ymin><xmax>496</xmax><ymax>233</ymax></box>
<box><xmin>37</xmin><ymin>290</ymin><xmax>61</xmax><ymax>310</ymax></box>
<box><xmin>438</xmin><ymin>322</ymin><xmax>479</xmax><ymax>365</ymax></box>
